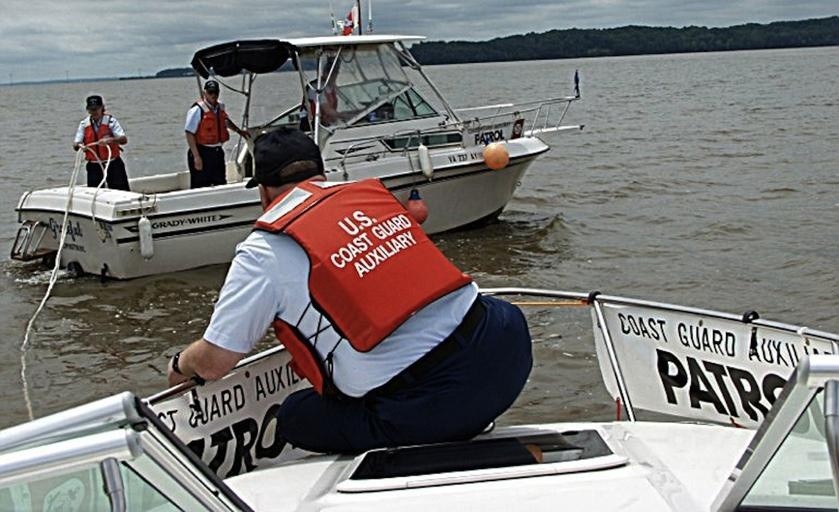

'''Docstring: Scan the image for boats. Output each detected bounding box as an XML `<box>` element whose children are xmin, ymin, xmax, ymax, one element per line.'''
<box><xmin>2</xmin><ymin>263</ymin><xmax>835</xmax><ymax>509</ymax></box>
<box><xmin>12</xmin><ymin>2</ymin><xmax>590</xmax><ymax>280</ymax></box>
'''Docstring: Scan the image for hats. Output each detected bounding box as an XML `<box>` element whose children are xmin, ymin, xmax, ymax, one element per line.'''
<box><xmin>204</xmin><ymin>79</ymin><xmax>220</xmax><ymax>92</ymax></box>
<box><xmin>244</xmin><ymin>128</ymin><xmax>324</xmax><ymax>188</ymax></box>
<box><xmin>85</xmin><ymin>94</ymin><xmax>103</xmax><ymax>111</ymax></box>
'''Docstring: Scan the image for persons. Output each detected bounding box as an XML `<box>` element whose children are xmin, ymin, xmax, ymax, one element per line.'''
<box><xmin>299</xmin><ymin>56</ymin><xmax>350</xmax><ymax>127</ymax></box>
<box><xmin>73</xmin><ymin>95</ymin><xmax>132</xmax><ymax>192</ymax></box>
<box><xmin>166</xmin><ymin>125</ymin><xmax>533</xmax><ymax>454</ymax></box>
<box><xmin>185</xmin><ymin>79</ymin><xmax>248</xmax><ymax>189</ymax></box>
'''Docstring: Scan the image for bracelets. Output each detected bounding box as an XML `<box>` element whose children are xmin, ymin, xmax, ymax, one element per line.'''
<box><xmin>172</xmin><ymin>351</ymin><xmax>183</xmax><ymax>376</ymax></box>
